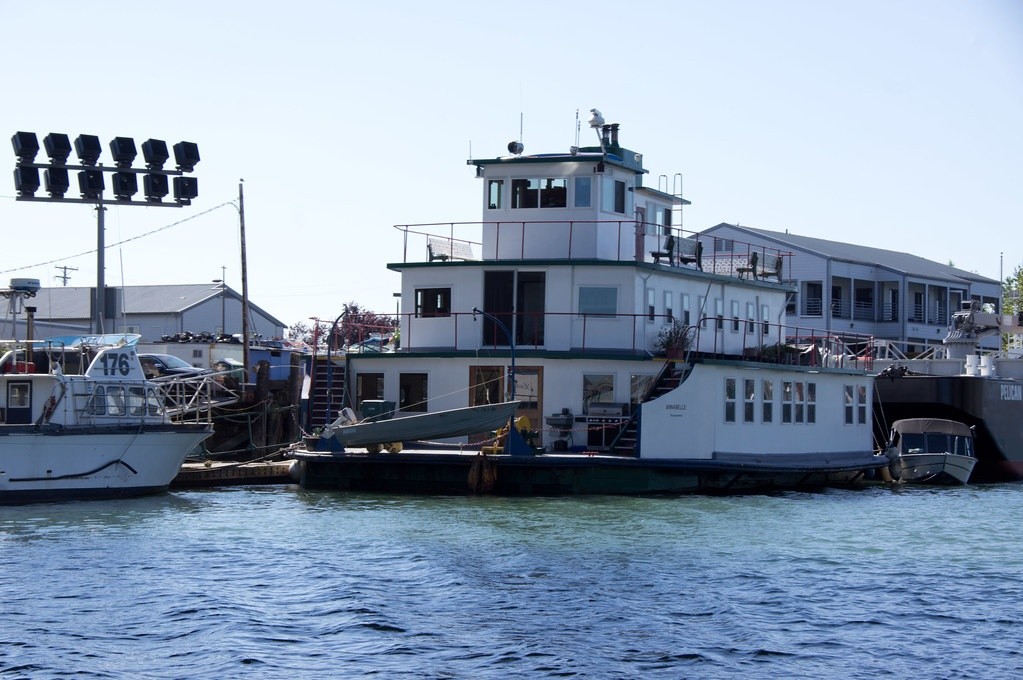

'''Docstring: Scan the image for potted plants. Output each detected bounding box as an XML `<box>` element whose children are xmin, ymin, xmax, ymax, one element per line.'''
<box><xmin>654</xmin><ymin>316</ymin><xmax>697</xmax><ymax>358</ymax></box>
<box><xmin>390</xmin><ymin>328</ymin><xmax>400</xmax><ymax>351</ymax></box>
<box><xmin>1003</xmin><ymin>297</ymin><xmax>1019</xmax><ymax>325</ymax></box>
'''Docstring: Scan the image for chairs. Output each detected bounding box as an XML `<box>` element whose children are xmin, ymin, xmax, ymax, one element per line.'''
<box><xmin>680</xmin><ymin>242</ymin><xmax>703</xmax><ymax>272</ymax></box>
<box><xmin>651</xmin><ymin>234</ymin><xmax>674</xmax><ymax>267</ymax></box>
<box><xmin>736</xmin><ymin>251</ymin><xmax>758</xmax><ymax>280</ymax></box>
<box><xmin>764</xmin><ymin>256</ymin><xmax>783</xmax><ymax>283</ymax></box>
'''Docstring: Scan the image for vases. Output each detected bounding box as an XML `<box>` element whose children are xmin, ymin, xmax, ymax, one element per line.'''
<box><xmin>744</xmin><ymin>347</ymin><xmax>756</xmax><ymax>358</ymax></box>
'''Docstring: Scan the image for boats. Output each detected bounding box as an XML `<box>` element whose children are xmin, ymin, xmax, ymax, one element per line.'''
<box><xmin>0</xmin><ymin>332</ymin><xmax>214</xmax><ymax>500</ymax></box>
<box><xmin>888</xmin><ymin>414</ymin><xmax>978</xmax><ymax>486</ymax></box>
<box><xmin>841</xmin><ymin>297</ymin><xmax>1023</xmax><ymax>484</ymax></box>
<box><xmin>279</xmin><ymin>106</ymin><xmax>889</xmax><ymax>498</ymax></box>
<box><xmin>332</xmin><ymin>400</ymin><xmax>521</xmax><ymax>447</ymax></box>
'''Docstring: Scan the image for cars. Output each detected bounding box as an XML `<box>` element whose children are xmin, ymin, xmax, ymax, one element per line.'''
<box><xmin>137</xmin><ymin>354</ymin><xmax>214</xmax><ymax>389</ymax></box>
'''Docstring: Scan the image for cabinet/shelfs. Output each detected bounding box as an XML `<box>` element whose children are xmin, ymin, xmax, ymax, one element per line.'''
<box><xmin>587</xmin><ymin>416</ymin><xmax>622</xmax><ymax>451</ymax></box>
